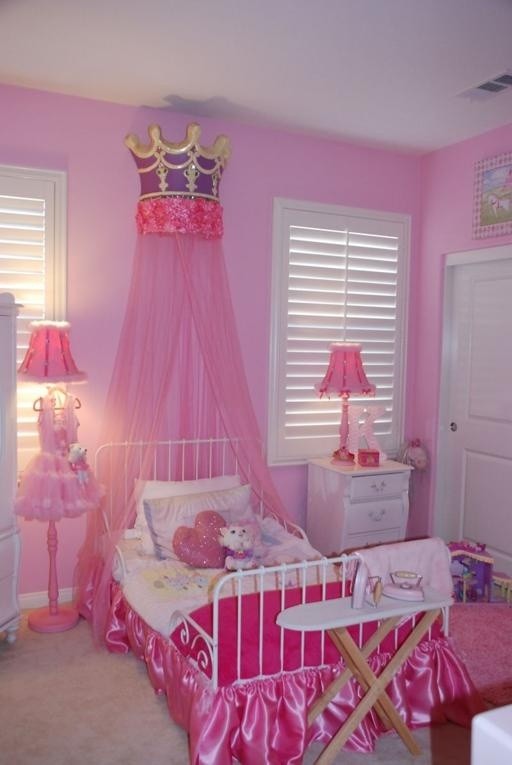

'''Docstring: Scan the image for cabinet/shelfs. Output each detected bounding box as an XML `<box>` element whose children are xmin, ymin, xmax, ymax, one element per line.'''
<box><xmin>304</xmin><ymin>458</ymin><xmax>415</xmax><ymax>562</ymax></box>
<box><xmin>1</xmin><ymin>289</ymin><xmax>21</xmax><ymax>646</ymax></box>
<box><xmin>33</xmin><ymin>381</ymin><xmax>83</xmax><ymax>412</ymax></box>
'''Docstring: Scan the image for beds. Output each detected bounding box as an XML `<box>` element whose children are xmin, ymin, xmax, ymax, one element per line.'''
<box><xmin>88</xmin><ymin>437</ymin><xmax>453</xmax><ymax>765</ymax></box>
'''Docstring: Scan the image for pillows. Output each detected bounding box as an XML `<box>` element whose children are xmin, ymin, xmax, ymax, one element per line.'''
<box><xmin>135</xmin><ymin>474</ymin><xmax>254</xmax><ymax>562</ymax></box>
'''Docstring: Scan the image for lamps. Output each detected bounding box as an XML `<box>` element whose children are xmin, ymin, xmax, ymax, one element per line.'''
<box><xmin>17</xmin><ymin>320</ymin><xmax>85</xmax><ymax>383</ymax></box>
<box><xmin>314</xmin><ymin>345</ymin><xmax>376</xmax><ymax>465</ymax></box>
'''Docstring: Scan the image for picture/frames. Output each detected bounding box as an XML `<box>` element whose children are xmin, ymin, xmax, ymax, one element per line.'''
<box><xmin>470</xmin><ymin>149</ymin><xmax>512</xmax><ymax>238</ymax></box>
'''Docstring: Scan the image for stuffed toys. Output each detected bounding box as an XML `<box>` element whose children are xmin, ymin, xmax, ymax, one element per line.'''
<box><xmin>396</xmin><ymin>438</ymin><xmax>426</xmax><ymax>472</ymax></box>
<box><xmin>69</xmin><ymin>441</ymin><xmax>91</xmax><ymax>486</ymax></box>
<box><xmin>217</xmin><ymin>516</ymin><xmax>269</xmax><ymax>571</ymax></box>
